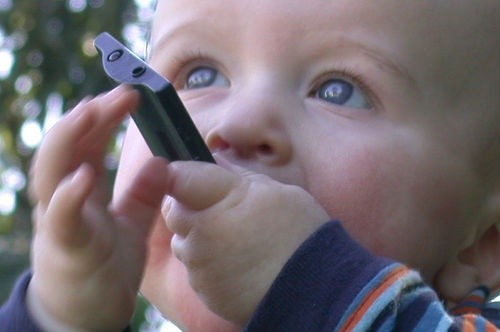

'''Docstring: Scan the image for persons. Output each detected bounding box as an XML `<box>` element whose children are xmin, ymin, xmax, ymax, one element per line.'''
<box><xmin>0</xmin><ymin>0</ymin><xmax>500</xmax><ymax>332</ymax></box>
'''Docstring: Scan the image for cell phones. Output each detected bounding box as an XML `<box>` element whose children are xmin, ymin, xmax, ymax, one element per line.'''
<box><xmin>93</xmin><ymin>34</ymin><xmax>217</xmax><ymax>164</ymax></box>
